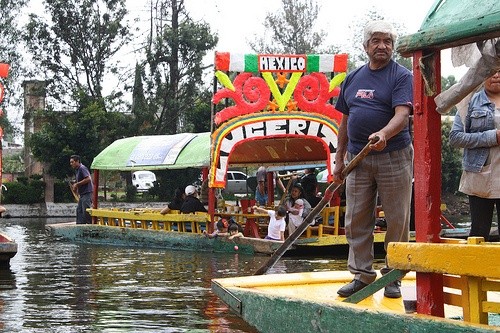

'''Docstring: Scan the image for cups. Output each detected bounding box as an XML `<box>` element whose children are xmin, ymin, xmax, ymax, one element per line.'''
<box><xmin>234</xmin><ymin>206</ymin><xmax>238</xmax><ymax>213</ymax></box>
<box><xmin>227</xmin><ymin>206</ymin><xmax>231</xmax><ymax>214</ymax></box>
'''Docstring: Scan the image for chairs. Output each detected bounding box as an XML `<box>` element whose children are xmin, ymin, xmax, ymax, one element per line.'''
<box><xmin>98</xmin><ymin>208</ymin><xmax>208</xmax><ymax>233</ymax></box>
<box><xmin>442</xmin><ymin>236</ymin><xmax>500</xmax><ymax>325</ymax></box>
<box><xmin>306</xmin><ymin>206</ymin><xmax>342</xmax><ymax>237</ymax></box>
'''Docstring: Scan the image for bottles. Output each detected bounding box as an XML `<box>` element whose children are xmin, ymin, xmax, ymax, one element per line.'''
<box><xmin>248</xmin><ymin>200</ymin><xmax>251</xmax><ymax>214</ymax></box>
<box><xmin>256</xmin><ymin>201</ymin><xmax>260</xmax><ymax>207</ymax></box>
<box><xmin>236</xmin><ymin>201</ymin><xmax>239</xmax><ymax>213</ymax></box>
<box><xmin>217</xmin><ymin>189</ymin><xmax>223</xmax><ymax>213</ymax></box>
<box><xmin>271</xmin><ymin>202</ymin><xmax>274</xmax><ymax>210</ymax></box>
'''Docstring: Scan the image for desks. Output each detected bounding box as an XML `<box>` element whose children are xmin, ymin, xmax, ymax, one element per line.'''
<box><xmin>215</xmin><ymin>213</ymin><xmax>269</xmax><ymax>238</ymax></box>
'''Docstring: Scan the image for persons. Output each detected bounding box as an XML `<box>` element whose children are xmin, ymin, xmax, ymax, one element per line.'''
<box><xmin>282</xmin><ymin>186</ymin><xmax>315</xmax><ymax>237</ymax></box>
<box><xmin>161</xmin><ymin>185</ymin><xmax>208</xmax><ymax>226</ymax></box>
<box><xmin>333</xmin><ymin>18</ymin><xmax>415</xmax><ymax>297</ymax></box>
<box><xmin>70</xmin><ymin>155</ymin><xmax>94</xmax><ymax>223</ymax></box>
<box><xmin>254</xmin><ymin>166</ymin><xmax>276</xmax><ymax>205</ymax></box>
<box><xmin>299</xmin><ymin>166</ymin><xmax>317</xmax><ymax>206</ymax></box>
<box><xmin>202</xmin><ymin>218</ymin><xmax>244</xmax><ymax>240</ymax></box>
<box><xmin>252</xmin><ymin>206</ymin><xmax>286</xmax><ymax>241</ymax></box>
<box><xmin>448</xmin><ymin>72</ymin><xmax>500</xmax><ymax>244</ymax></box>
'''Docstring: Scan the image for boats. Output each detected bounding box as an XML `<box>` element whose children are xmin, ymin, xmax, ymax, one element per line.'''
<box><xmin>211</xmin><ymin>0</ymin><xmax>500</xmax><ymax>333</ymax></box>
<box><xmin>45</xmin><ymin>51</ymin><xmax>416</xmax><ymax>256</ymax></box>
<box><xmin>0</xmin><ymin>63</ymin><xmax>18</xmax><ymax>263</ymax></box>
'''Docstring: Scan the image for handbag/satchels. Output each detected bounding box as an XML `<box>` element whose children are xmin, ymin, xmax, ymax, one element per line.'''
<box><xmin>301</xmin><ymin>199</ymin><xmax>311</xmax><ymax>218</ymax></box>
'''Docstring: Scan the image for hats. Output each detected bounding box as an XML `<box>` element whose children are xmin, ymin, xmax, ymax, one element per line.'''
<box><xmin>185</xmin><ymin>185</ymin><xmax>195</xmax><ymax>195</ymax></box>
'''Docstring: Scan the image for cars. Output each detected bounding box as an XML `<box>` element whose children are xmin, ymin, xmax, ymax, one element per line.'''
<box><xmin>224</xmin><ymin>171</ymin><xmax>253</xmax><ymax>194</ymax></box>
<box><xmin>133</xmin><ymin>171</ymin><xmax>156</xmax><ymax>194</ymax></box>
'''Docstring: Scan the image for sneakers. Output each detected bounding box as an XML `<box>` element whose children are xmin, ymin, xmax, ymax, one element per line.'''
<box><xmin>337</xmin><ymin>278</ymin><xmax>366</xmax><ymax>296</ymax></box>
<box><xmin>384</xmin><ymin>280</ymin><xmax>401</xmax><ymax>298</ymax></box>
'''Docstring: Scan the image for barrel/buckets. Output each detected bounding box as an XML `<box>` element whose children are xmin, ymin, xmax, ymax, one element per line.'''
<box><xmin>240</xmin><ymin>199</ymin><xmax>256</xmax><ymax>214</ymax></box>
